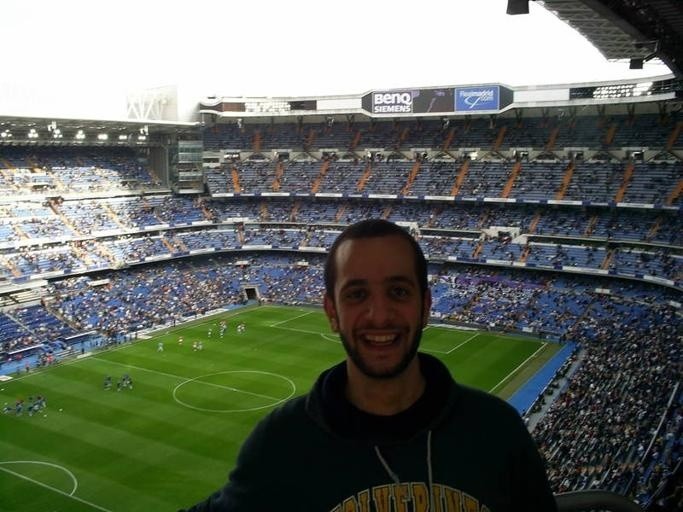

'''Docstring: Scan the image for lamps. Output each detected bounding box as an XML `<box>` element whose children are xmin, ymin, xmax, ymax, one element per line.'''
<box><xmin>506</xmin><ymin>0</ymin><xmax>529</xmax><ymax>15</ymax></box>
<box><xmin>630</xmin><ymin>39</ymin><xmax>663</xmax><ymax>69</ymax></box>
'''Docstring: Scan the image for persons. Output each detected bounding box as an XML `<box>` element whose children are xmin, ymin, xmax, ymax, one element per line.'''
<box><xmin>188</xmin><ymin>220</ymin><xmax>558</xmax><ymax>511</ymax></box>
<box><xmin>2</xmin><ymin>112</ymin><xmax>249</xmax><ymax>418</ymax></box>
<box><xmin>248</xmin><ymin>114</ymin><xmax>683</xmax><ymax>510</ymax></box>
